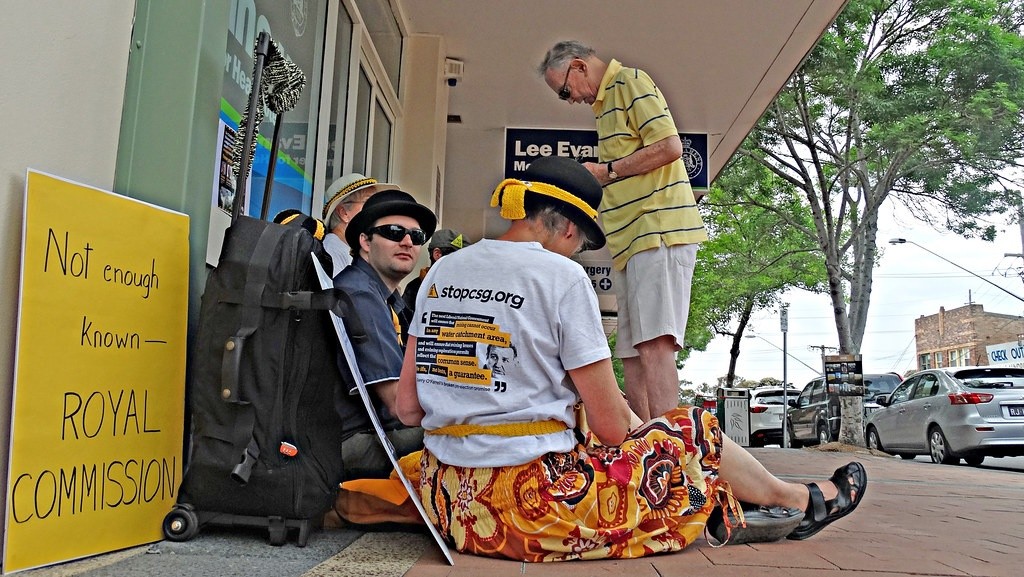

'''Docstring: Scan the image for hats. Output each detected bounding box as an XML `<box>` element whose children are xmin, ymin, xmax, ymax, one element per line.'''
<box><xmin>322</xmin><ymin>173</ymin><xmax>400</xmax><ymax>228</ymax></box>
<box><xmin>490</xmin><ymin>156</ymin><xmax>606</xmax><ymax>253</ymax></box>
<box><xmin>273</xmin><ymin>208</ymin><xmax>316</xmax><ymax>236</ymax></box>
<box><xmin>428</xmin><ymin>229</ymin><xmax>472</xmax><ymax>250</ymax></box>
<box><xmin>345</xmin><ymin>189</ymin><xmax>437</xmax><ymax>250</ymax></box>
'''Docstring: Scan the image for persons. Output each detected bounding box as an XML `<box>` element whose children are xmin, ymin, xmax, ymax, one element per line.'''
<box><xmin>530</xmin><ymin>40</ymin><xmax>709</xmax><ymax>421</ymax></box>
<box><xmin>395</xmin><ymin>155</ymin><xmax>867</xmax><ymax>563</ymax></box>
<box><xmin>318</xmin><ymin>173</ymin><xmax>474</xmax><ymax>482</ymax></box>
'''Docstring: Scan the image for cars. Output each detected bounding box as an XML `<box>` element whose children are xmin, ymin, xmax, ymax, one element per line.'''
<box><xmin>863</xmin><ymin>365</ymin><xmax>1024</xmax><ymax>468</ymax></box>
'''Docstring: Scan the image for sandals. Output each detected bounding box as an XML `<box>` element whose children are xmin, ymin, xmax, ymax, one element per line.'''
<box><xmin>707</xmin><ymin>500</ymin><xmax>806</xmax><ymax>544</ymax></box>
<box><xmin>785</xmin><ymin>462</ymin><xmax>867</xmax><ymax>540</ymax></box>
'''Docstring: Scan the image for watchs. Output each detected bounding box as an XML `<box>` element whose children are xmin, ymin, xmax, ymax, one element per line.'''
<box><xmin>603</xmin><ymin>162</ymin><xmax>621</xmax><ymax>180</ymax></box>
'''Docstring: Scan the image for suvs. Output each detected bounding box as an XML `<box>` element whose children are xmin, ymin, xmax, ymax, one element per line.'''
<box><xmin>782</xmin><ymin>372</ymin><xmax>906</xmax><ymax>450</ymax></box>
<box><xmin>747</xmin><ymin>385</ymin><xmax>808</xmax><ymax>448</ymax></box>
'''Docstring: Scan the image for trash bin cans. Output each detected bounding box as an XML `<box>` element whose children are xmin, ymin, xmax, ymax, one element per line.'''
<box><xmin>716</xmin><ymin>387</ymin><xmax>751</xmax><ymax>447</ymax></box>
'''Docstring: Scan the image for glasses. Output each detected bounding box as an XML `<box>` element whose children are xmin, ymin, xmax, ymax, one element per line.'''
<box><xmin>558</xmin><ymin>56</ymin><xmax>579</xmax><ymax>100</ymax></box>
<box><xmin>368</xmin><ymin>224</ymin><xmax>427</xmax><ymax>245</ymax></box>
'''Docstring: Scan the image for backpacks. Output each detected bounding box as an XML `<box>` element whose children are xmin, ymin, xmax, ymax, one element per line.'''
<box><xmin>161</xmin><ymin>215</ymin><xmax>368</xmax><ymax>546</ymax></box>
